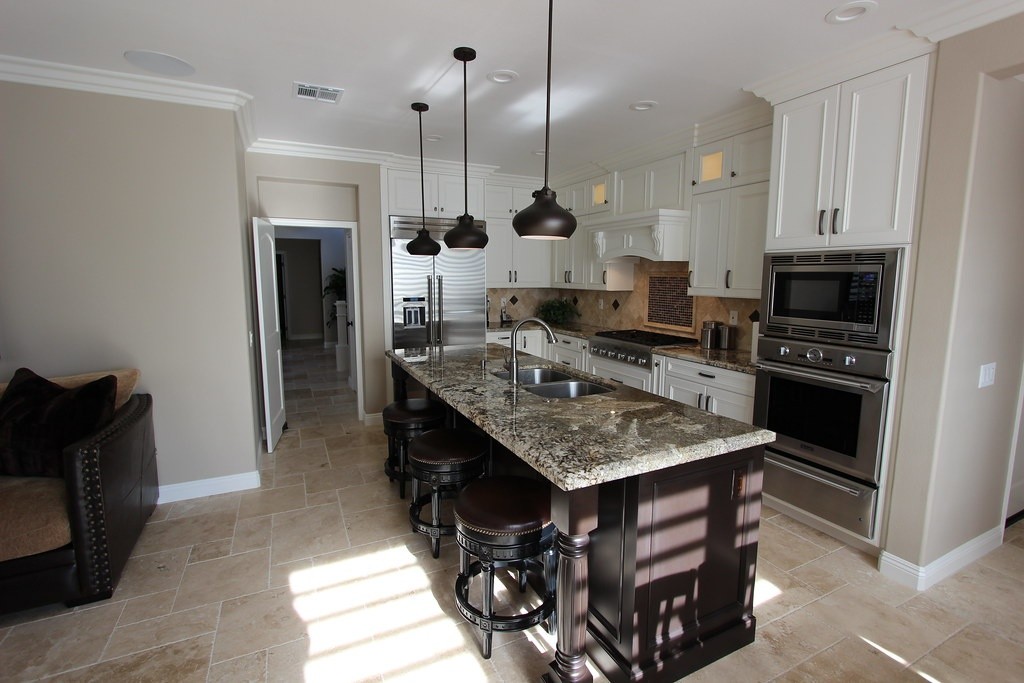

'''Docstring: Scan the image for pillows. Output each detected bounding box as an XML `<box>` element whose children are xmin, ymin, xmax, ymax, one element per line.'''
<box><xmin>0</xmin><ymin>366</ymin><xmax>140</xmax><ymax>411</ymax></box>
<box><xmin>0</xmin><ymin>366</ymin><xmax>118</xmax><ymax>477</ymax></box>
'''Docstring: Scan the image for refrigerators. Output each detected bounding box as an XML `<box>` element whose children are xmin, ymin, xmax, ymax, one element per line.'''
<box><xmin>390</xmin><ymin>236</ymin><xmax>487</xmax><ymax>407</ymax></box>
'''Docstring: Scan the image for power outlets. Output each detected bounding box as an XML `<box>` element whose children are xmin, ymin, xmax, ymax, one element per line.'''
<box><xmin>598</xmin><ymin>299</ymin><xmax>603</xmax><ymax>309</ymax></box>
<box><xmin>501</xmin><ymin>297</ymin><xmax>506</xmax><ymax>306</ymax></box>
<box><xmin>729</xmin><ymin>311</ymin><xmax>740</xmax><ymax>325</ymax></box>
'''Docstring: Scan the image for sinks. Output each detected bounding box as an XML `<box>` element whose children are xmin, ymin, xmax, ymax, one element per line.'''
<box><xmin>490</xmin><ymin>368</ymin><xmax>572</xmax><ymax>384</ymax></box>
<box><xmin>521</xmin><ymin>383</ymin><xmax>617</xmax><ymax>398</ymax></box>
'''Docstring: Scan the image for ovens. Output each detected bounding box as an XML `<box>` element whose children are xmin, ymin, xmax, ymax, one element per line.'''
<box><xmin>741</xmin><ymin>248</ymin><xmax>908</xmax><ymax>540</ymax></box>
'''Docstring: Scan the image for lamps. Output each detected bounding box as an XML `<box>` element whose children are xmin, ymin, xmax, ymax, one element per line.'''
<box><xmin>511</xmin><ymin>0</ymin><xmax>578</xmax><ymax>242</ymax></box>
<box><xmin>406</xmin><ymin>102</ymin><xmax>442</xmax><ymax>257</ymax></box>
<box><xmin>443</xmin><ymin>46</ymin><xmax>490</xmax><ymax>248</ymax></box>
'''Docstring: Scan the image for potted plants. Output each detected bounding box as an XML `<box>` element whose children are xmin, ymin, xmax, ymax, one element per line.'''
<box><xmin>537</xmin><ymin>297</ymin><xmax>582</xmax><ymax>328</ymax></box>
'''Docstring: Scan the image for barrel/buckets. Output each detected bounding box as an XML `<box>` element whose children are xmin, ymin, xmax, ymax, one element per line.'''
<box><xmin>701</xmin><ymin>321</ymin><xmax>736</xmax><ymax>349</ymax></box>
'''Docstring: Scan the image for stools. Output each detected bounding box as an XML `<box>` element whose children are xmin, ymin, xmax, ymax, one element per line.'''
<box><xmin>382</xmin><ymin>398</ymin><xmax>446</xmax><ymax>500</ymax></box>
<box><xmin>453</xmin><ymin>474</ymin><xmax>558</xmax><ymax>659</ymax></box>
<box><xmin>407</xmin><ymin>426</ymin><xmax>487</xmax><ymax>559</ymax></box>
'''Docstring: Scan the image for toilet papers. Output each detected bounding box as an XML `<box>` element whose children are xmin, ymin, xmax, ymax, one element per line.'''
<box><xmin>751</xmin><ymin>321</ymin><xmax>759</xmax><ymax>363</ymax></box>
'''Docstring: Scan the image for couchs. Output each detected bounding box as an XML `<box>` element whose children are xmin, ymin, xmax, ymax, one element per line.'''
<box><xmin>0</xmin><ymin>392</ymin><xmax>160</xmax><ymax>608</ymax></box>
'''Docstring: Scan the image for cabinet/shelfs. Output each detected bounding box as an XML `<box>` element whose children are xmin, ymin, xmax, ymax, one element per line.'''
<box><xmin>589</xmin><ymin>354</ymin><xmax>651</xmax><ymax>393</ymax></box>
<box><xmin>522</xmin><ymin>331</ymin><xmax>542</xmax><ymax>359</ymax></box>
<box><xmin>764</xmin><ymin>53</ymin><xmax>931</xmax><ymax>254</ymax></box>
<box><xmin>484</xmin><ymin>182</ymin><xmax>538</xmax><ymax>218</ymax></box>
<box><xmin>588</xmin><ymin>230</ymin><xmax>608</xmax><ymax>291</ymax></box>
<box><xmin>651</xmin><ymin>352</ymin><xmax>665</xmax><ymax>397</ymax></box>
<box><xmin>487</xmin><ymin>331</ymin><xmax>521</xmax><ymax>352</ymax></box>
<box><xmin>693</xmin><ymin>124</ymin><xmax>773</xmax><ymax>194</ymax></box>
<box><xmin>688</xmin><ymin>181</ymin><xmax>769</xmax><ymax>300</ymax></box>
<box><xmin>580</xmin><ymin>340</ymin><xmax>590</xmax><ymax>372</ymax></box>
<box><xmin>486</xmin><ymin>217</ymin><xmax>550</xmax><ymax>289</ymax></box>
<box><xmin>554</xmin><ymin>179</ymin><xmax>585</xmax><ymax>216</ymax></box>
<box><xmin>665</xmin><ymin>357</ymin><xmax>756</xmax><ymax>425</ymax></box>
<box><xmin>585</xmin><ymin>175</ymin><xmax>611</xmax><ymax>215</ymax></box>
<box><xmin>545</xmin><ymin>331</ymin><xmax>582</xmax><ymax>371</ymax></box>
<box><xmin>551</xmin><ymin>227</ymin><xmax>586</xmax><ymax>288</ymax></box>
<box><xmin>387</xmin><ymin>168</ymin><xmax>485</xmax><ymax>220</ymax></box>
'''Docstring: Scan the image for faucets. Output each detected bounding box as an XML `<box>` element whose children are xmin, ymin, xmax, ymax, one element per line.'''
<box><xmin>509</xmin><ymin>317</ymin><xmax>558</xmax><ymax>385</ymax></box>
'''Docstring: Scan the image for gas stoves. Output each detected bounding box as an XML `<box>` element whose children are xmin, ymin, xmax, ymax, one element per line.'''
<box><xmin>587</xmin><ymin>329</ymin><xmax>699</xmax><ymax>372</ymax></box>
